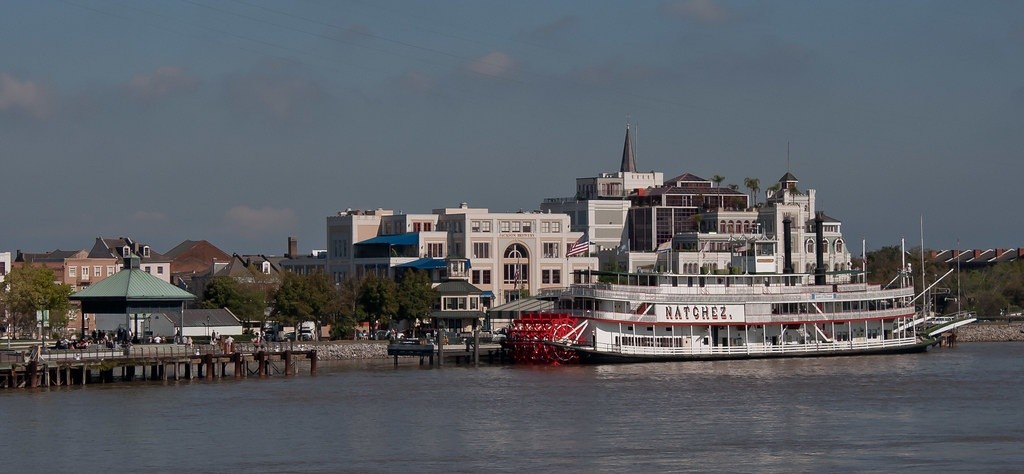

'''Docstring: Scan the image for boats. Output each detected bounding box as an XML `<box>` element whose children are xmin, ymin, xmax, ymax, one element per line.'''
<box><xmin>533</xmin><ymin>142</ymin><xmax>976</xmax><ymax>361</ymax></box>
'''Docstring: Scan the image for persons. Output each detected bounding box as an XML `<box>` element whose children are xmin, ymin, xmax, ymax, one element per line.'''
<box><xmin>358</xmin><ymin>325</ymin><xmax>399</xmax><ymax>341</ymax></box>
<box><xmin>54</xmin><ymin>327</ymin><xmax>265</xmax><ymax>349</ymax></box>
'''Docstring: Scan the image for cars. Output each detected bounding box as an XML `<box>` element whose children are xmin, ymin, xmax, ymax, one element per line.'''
<box><xmin>370</xmin><ymin>330</ymin><xmax>387</xmax><ymax>340</ymax></box>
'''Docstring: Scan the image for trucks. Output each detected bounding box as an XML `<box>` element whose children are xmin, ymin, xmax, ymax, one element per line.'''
<box><xmin>297</xmin><ymin>320</ymin><xmax>315</xmax><ymax>343</ymax></box>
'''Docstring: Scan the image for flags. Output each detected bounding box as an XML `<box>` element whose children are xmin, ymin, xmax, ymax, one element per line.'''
<box><xmin>565</xmin><ymin>232</ymin><xmax>589</xmax><ymax>257</ymax></box>
<box><xmin>514</xmin><ymin>260</ymin><xmax>520</xmax><ymax>287</ymax></box>
